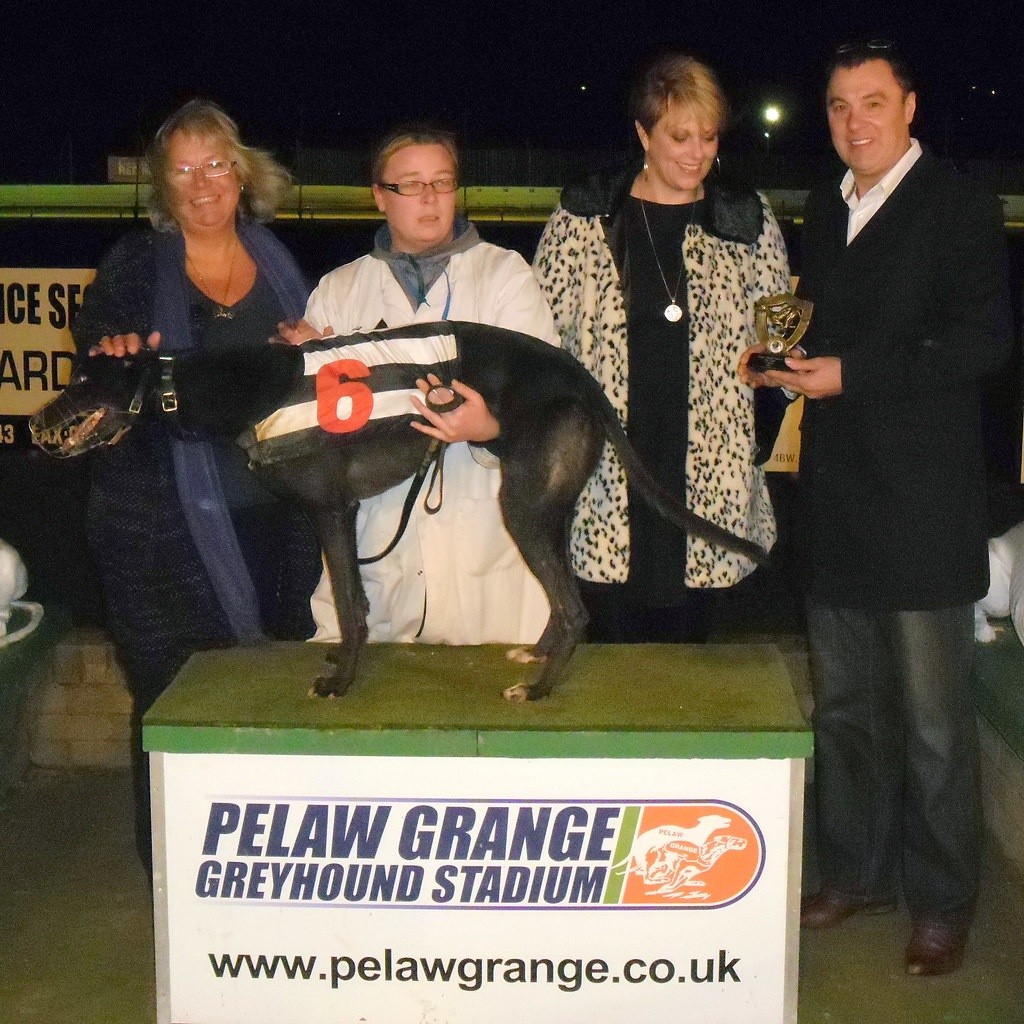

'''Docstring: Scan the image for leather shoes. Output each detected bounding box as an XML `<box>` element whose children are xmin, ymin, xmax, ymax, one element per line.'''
<box><xmin>904</xmin><ymin>920</ymin><xmax>968</xmax><ymax>975</ymax></box>
<box><xmin>801</xmin><ymin>883</ymin><xmax>897</xmax><ymax>928</ymax></box>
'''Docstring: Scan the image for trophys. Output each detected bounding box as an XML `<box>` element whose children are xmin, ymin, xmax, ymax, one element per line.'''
<box><xmin>749</xmin><ymin>293</ymin><xmax>814</xmax><ymax>372</ymax></box>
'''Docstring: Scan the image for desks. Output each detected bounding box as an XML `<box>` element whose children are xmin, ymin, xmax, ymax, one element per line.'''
<box><xmin>139</xmin><ymin>640</ymin><xmax>818</xmax><ymax>1024</ymax></box>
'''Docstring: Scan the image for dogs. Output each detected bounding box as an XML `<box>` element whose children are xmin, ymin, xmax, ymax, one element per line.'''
<box><xmin>29</xmin><ymin>319</ymin><xmax>768</xmax><ymax>705</ymax></box>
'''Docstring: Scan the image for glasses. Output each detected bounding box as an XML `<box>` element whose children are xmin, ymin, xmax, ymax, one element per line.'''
<box><xmin>377</xmin><ymin>176</ymin><xmax>460</xmax><ymax>196</ymax></box>
<box><xmin>165</xmin><ymin>160</ymin><xmax>238</xmax><ymax>183</ymax></box>
<box><xmin>834</xmin><ymin>39</ymin><xmax>897</xmax><ymax>55</ymax></box>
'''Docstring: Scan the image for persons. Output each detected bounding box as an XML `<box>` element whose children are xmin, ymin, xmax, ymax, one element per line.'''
<box><xmin>736</xmin><ymin>42</ymin><xmax>1023</xmax><ymax>975</ymax></box>
<box><xmin>72</xmin><ymin>100</ymin><xmax>323</xmax><ymax>870</ymax></box>
<box><xmin>266</xmin><ymin>127</ymin><xmax>561</xmax><ymax>647</ymax></box>
<box><xmin>540</xmin><ymin>56</ymin><xmax>789</xmax><ymax>643</ymax></box>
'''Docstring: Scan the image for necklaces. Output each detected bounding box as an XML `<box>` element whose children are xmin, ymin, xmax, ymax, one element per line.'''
<box><xmin>185</xmin><ymin>234</ymin><xmax>239</xmax><ymax>320</ymax></box>
<box><xmin>637</xmin><ymin>173</ymin><xmax>698</xmax><ymax>323</ymax></box>
